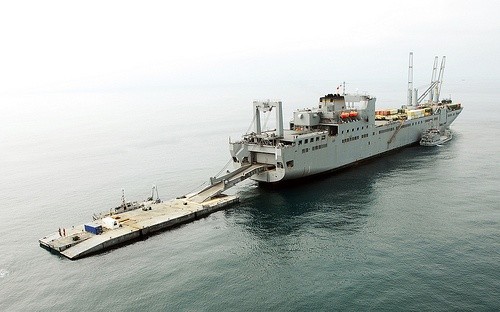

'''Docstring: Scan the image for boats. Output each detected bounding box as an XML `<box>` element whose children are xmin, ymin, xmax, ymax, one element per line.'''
<box><xmin>190</xmin><ymin>52</ymin><xmax>464</xmax><ymax>204</ymax></box>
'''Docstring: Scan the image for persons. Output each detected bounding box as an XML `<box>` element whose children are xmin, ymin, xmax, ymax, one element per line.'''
<box><xmin>59</xmin><ymin>227</ymin><xmax>62</xmax><ymax>236</ymax></box>
<box><xmin>63</xmin><ymin>228</ymin><xmax>65</xmax><ymax>236</ymax></box>
